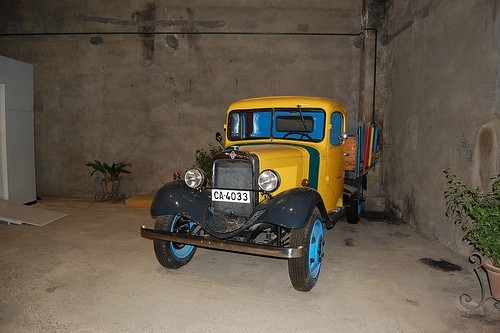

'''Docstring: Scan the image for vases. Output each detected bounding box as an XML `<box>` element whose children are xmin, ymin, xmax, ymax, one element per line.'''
<box><xmin>439</xmin><ymin>165</ymin><xmax>500</xmax><ymax>302</ymax></box>
<box><xmin>85</xmin><ymin>158</ymin><xmax>132</xmax><ymax>196</ymax></box>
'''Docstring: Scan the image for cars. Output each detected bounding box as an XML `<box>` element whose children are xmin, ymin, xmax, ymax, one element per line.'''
<box><xmin>139</xmin><ymin>95</ymin><xmax>385</xmax><ymax>292</ymax></box>
